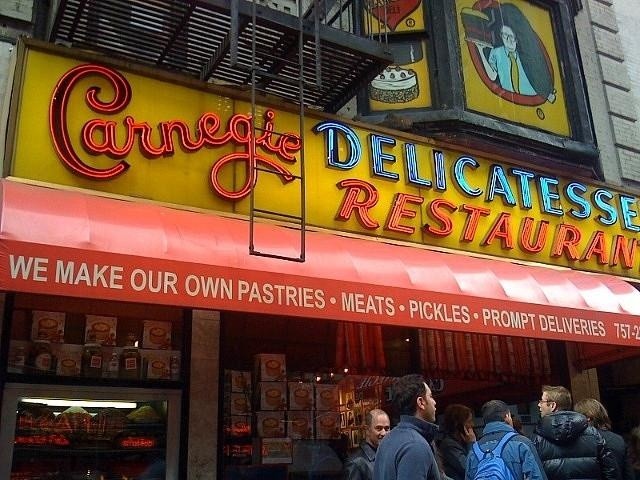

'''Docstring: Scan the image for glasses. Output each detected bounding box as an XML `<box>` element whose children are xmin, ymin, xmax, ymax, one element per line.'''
<box><xmin>537</xmin><ymin>399</ymin><xmax>554</xmax><ymax>404</ymax></box>
<box><xmin>501</xmin><ymin>33</ymin><xmax>518</xmax><ymax>42</ymax></box>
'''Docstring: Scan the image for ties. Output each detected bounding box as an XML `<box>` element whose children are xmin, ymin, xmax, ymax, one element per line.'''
<box><xmin>508</xmin><ymin>52</ymin><xmax>522</xmax><ymax>94</ymax></box>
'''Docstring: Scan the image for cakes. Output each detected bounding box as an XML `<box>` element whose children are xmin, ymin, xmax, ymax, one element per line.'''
<box><xmin>369</xmin><ymin>66</ymin><xmax>419</xmax><ymax>104</ymax></box>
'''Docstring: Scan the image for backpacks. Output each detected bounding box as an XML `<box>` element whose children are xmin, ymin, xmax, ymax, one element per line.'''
<box><xmin>469</xmin><ymin>432</ymin><xmax>519</xmax><ymax>478</ymax></box>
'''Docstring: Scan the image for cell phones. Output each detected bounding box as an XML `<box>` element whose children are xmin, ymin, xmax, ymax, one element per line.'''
<box><xmin>456</xmin><ymin>422</ymin><xmax>466</xmax><ymax>436</ymax></box>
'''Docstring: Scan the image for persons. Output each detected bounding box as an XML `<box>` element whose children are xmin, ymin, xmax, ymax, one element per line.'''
<box><xmin>570</xmin><ymin>397</ymin><xmax>629</xmax><ymax>480</ymax></box>
<box><xmin>430</xmin><ymin>402</ymin><xmax>478</xmax><ymax>480</ymax></box>
<box><xmin>343</xmin><ymin>407</ymin><xmax>392</xmax><ymax>480</ymax></box>
<box><xmin>372</xmin><ymin>374</ymin><xmax>454</xmax><ymax>480</ymax></box>
<box><xmin>464</xmin><ymin>399</ymin><xmax>548</xmax><ymax>480</ymax></box>
<box><xmin>525</xmin><ymin>384</ymin><xmax>625</xmax><ymax>480</ymax></box>
